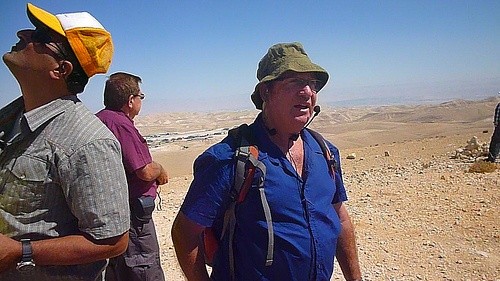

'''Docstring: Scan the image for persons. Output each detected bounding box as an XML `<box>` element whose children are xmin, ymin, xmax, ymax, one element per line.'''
<box><xmin>483</xmin><ymin>103</ymin><xmax>500</xmax><ymax>163</ymax></box>
<box><xmin>171</xmin><ymin>42</ymin><xmax>363</xmax><ymax>281</ymax></box>
<box><xmin>0</xmin><ymin>4</ymin><xmax>168</xmax><ymax>281</ymax></box>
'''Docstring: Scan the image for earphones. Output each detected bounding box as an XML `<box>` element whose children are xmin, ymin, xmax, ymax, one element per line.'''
<box><xmin>55</xmin><ymin>64</ymin><xmax>66</xmax><ymax>73</ymax></box>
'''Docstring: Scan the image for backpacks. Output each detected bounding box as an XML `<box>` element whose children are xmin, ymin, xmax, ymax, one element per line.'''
<box><xmin>198</xmin><ymin>124</ymin><xmax>338</xmax><ymax>270</ymax></box>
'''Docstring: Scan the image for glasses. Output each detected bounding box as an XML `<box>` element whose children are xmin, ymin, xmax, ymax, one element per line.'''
<box><xmin>278</xmin><ymin>76</ymin><xmax>325</xmax><ymax>91</ymax></box>
<box><xmin>128</xmin><ymin>93</ymin><xmax>144</xmax><ymax>100</ymax></box>
<box><xmin>31</xmin><ymin>27</ymin><xmax>71</xmax><ymax>63</ymax></box>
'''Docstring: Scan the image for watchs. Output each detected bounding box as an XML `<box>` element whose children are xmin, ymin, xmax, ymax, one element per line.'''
<box><xmin>16</xmin><ymin>239</ymin><xmax>36</xmax><ymax>274</ymax></box>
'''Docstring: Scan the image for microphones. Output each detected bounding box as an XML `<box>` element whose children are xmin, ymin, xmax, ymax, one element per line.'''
<box><xmin>299</xmin><ymin>105</ymin><xmax>321</xmax><ymax>134</ymax></box>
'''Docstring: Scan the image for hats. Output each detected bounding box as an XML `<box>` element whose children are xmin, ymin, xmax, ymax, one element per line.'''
<box><xmin>26</xmin><ymin>2</ymin><xmax>114</xmax><ymax>77</ymax></box>
<box><xmin>250</xmin><ymin>41</ymin><xmax>330</xmax><ymax>111</ymax></box>
